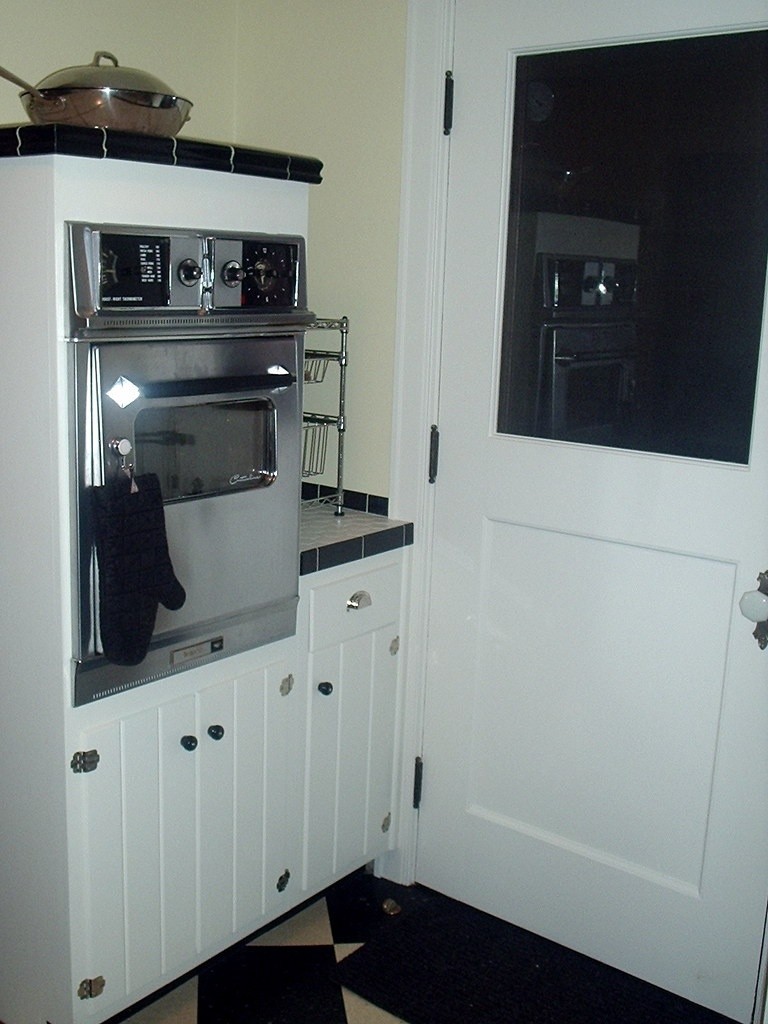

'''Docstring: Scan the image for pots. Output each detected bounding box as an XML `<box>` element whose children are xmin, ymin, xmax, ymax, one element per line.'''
<box><xmin>0</xmin><ymin>50</ymin><xmax>195</xmax><ymax>138</ymax></box>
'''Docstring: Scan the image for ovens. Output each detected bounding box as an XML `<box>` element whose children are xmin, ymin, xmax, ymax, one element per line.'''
<box><xmin>68</xmin><ymin>225</ymin><xmax>318</xmax><ymax>708</ymax></box>
<box><xmin>528</xmin><ymin>251</ymin><xmax>641</xmax><ymax>443</ymax></box>
